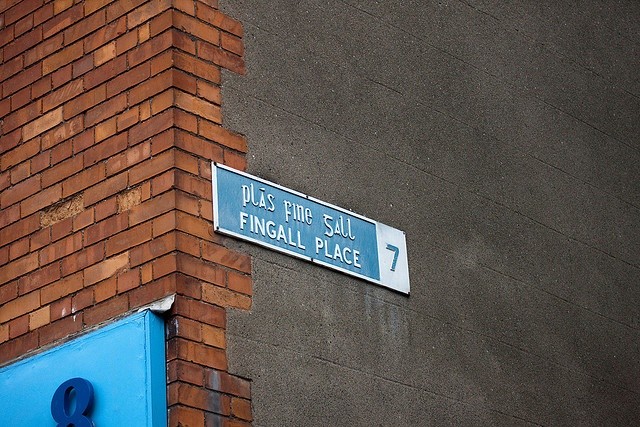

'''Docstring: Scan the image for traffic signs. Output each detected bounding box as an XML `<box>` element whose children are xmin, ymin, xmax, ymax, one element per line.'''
<box><xmin>211</xmin><ymin>160</ymin><xmax>411</xmax><ymax>297</ymax></box>
